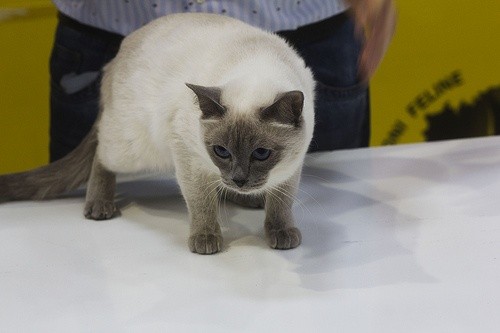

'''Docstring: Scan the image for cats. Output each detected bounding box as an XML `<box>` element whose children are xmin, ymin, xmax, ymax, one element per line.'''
<box><xmin>0</xmin><ymin>11</ymin><xmax>318</xmax><ymax>256</ymax></box>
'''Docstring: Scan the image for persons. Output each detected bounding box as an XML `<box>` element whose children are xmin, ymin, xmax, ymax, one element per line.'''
<box><xmin>48</xmin><ymin>0</ymin><xmax>397</xmax><ymax>164</ymax></box>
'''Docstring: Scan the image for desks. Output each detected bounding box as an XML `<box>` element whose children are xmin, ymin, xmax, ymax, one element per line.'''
<box><xmin>0</xmin><ymin>136</ymin><xmax>500</xmax><ymax>333</ymax></box>
